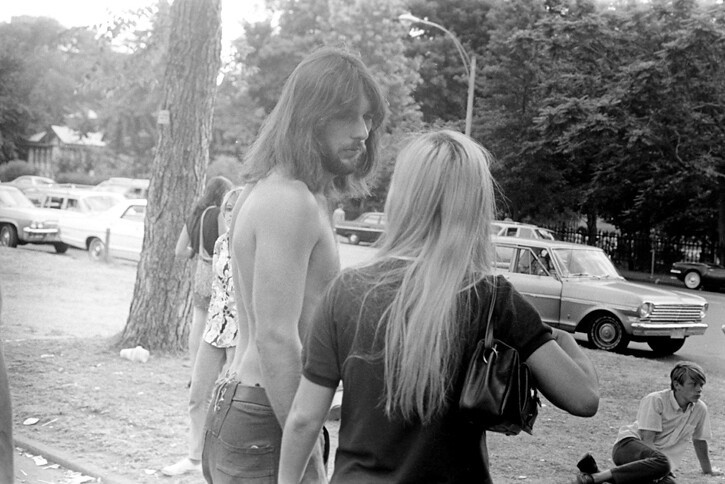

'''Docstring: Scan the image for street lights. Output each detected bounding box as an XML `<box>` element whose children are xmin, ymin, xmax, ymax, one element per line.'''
<box><xmin>398</xmin><ymin>13</ymin><xmax>477</xmax><ymax>136</ymax></box>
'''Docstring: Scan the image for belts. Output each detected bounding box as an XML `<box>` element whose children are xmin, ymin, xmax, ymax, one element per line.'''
<box><xmin>219</xmin><ymin>383</ymin><xmax>270</xmax><ymax>406</ymax></box>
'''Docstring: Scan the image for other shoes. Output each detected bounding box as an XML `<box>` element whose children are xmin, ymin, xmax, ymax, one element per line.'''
<box><xmin>163</xmin><ymin>458</ymin><xmax>204</xmax><ymax>475</ymax></box>
<box><xmin>577</xmin><ymin>473</ymin><xmax>595</xmax><ymax>484</ymax></box>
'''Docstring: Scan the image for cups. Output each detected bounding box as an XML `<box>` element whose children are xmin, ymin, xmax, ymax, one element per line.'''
<box><xmin>120</xmin><ymin>345</ymin><xmax>150</xmax><ymax>362</ymax></box>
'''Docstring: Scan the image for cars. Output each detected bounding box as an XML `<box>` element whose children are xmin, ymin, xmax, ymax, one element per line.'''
<box><xmin>487</xmin><ymin>219</ymin><xmax>574</xmax><ymax>267</ymax></box>
<box><xmin>334</xmin><ymin>210</ymin><xmax>386</xmax><ymax>245</ymax></box>
<box><xmin>668</xmin><ymin>260</ymin><xmax>725</xmax><ymax>293</ymax></box>
<box><xmin>0</xmin><ymin>170</ymin><xmax>151</xmax><ymax>263</ymax></box>
<box><xmin>488</xmin><ymin>233</ymin><xmax>709</xmax><ymax>356</ymax></box>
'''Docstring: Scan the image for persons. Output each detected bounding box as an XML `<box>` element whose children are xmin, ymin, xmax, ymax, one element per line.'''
<box><xmin>200</xmin><ymin>48</ymin><xmax>387</xmax><ymax>484</ymax></box>
<box><xmin>277</xmin><ymin>130</ymin><xmax>600</xmax><ymax>483</ymax></box>
<box><xmin>175</xmin><ymin>176</ymin><xmax>243</xmax><ymax>467</ymax></box>
<box><xmin>332</xmin><ymin>203</ymin><xmax>345</xmax><ymax>227</ymax></box>
<box><xmin>577</xmin><ymin>361</ymin><xmax>724</xmax><ymax>484</ymax></box>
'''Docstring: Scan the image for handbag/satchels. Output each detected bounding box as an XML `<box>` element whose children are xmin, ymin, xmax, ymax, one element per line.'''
<box><xmin>190</xmin><ymin>251</ymin><xmax>214</xmax><ymax>312</ymax></box>
<box><xmin>459</xmin><ymin>275</ymin><xmax>541</xmax><ymax>436</ymax></box>
